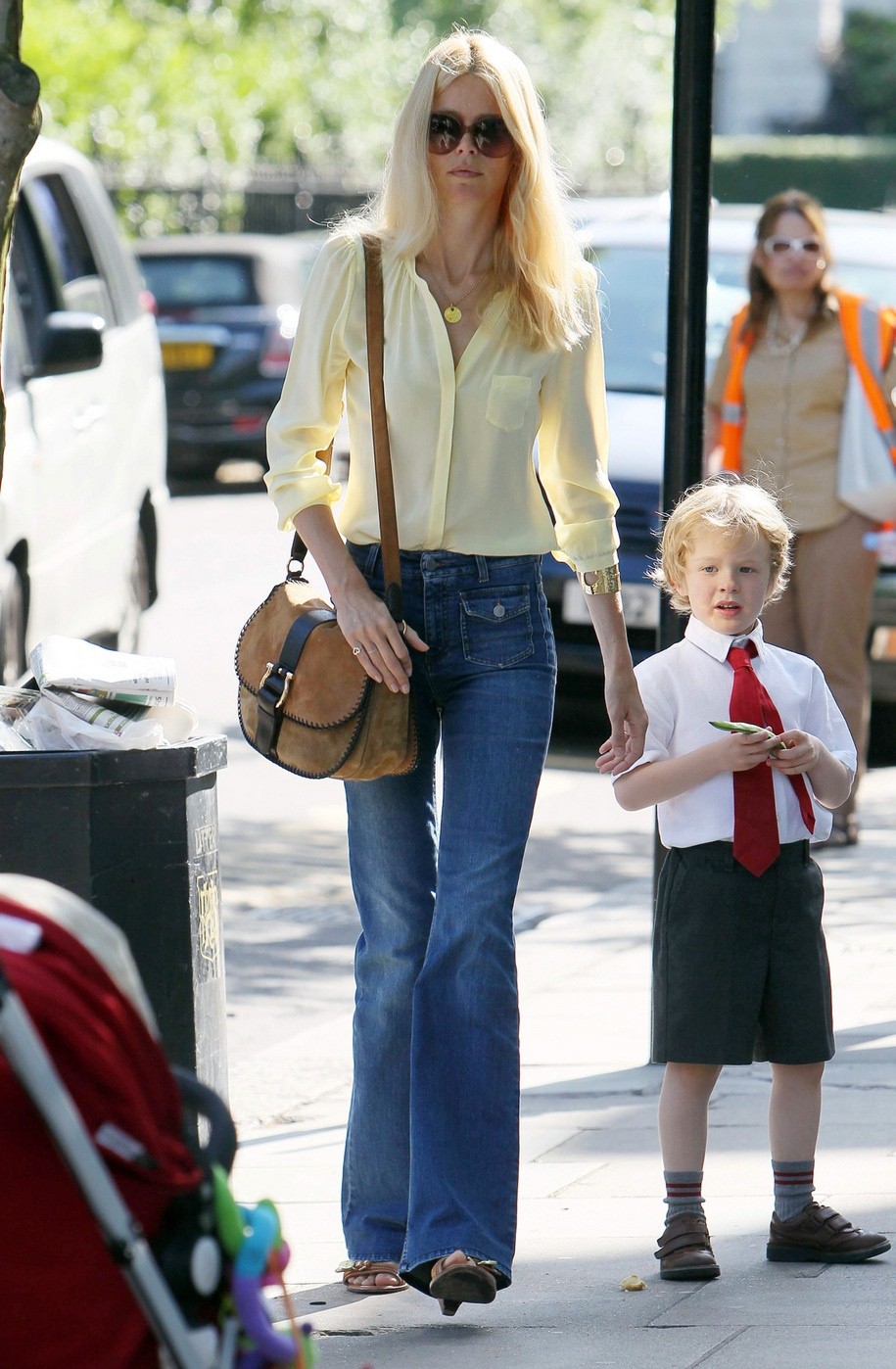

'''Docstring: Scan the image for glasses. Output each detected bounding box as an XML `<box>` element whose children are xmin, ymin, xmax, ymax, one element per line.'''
<box><xmin>427</xmin><ymin>113</ymin><xmax>514</xmax><ymax>159</ymax></box>
<box><xmin>762</xmin><ymin>235</ymin><xmax>822</xmax><ymax>260</ymax></box>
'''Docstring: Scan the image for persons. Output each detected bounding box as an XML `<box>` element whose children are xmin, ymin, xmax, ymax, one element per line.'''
<box><xmin>708</xmin><ymin>188</ymin><xmax>896</xmax><ymax>846</ymax></box>
<box><xmin>605</xmin><ymin>475</ymin><xmax>893</xmax><ymax>1282</ymax></box>
<box><xmin>263</xmin><ymin>23</ymin><xmax>651</xmax><ymax>1318</ymax></box>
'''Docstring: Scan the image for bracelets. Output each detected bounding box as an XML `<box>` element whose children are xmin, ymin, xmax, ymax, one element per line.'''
<box><xmin>579</xmin><ymin>564</ymin><xmax>621</xmax><ymax>595</ymax></box>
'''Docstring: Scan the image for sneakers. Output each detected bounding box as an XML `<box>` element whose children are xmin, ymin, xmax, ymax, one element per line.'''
<box><xmin>765</xmin><ymin>1201</ymin><xmax>891</xmax><ymax>1264</ymax></box>
<box><xmin>653</xmin><ymin>1212</ymin><xmax>720</xmax><ymax>1280</ymax></box>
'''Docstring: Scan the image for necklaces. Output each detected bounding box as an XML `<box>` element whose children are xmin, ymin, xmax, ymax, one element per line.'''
<box><xmin>766</xmin><ymin>308</ymin><xmax>814</xmax><ymax>356</ymax></box>
<box><xmin>422</xmin><ymin>248</ymin><xmax>490</xmax><ymax>324</ymax></box>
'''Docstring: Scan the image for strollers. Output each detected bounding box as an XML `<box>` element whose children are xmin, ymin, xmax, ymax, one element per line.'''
<box><xmin>0</xmin><ymin>876</ymin><xmax>310</xmax><ymax>1369</ymax></box>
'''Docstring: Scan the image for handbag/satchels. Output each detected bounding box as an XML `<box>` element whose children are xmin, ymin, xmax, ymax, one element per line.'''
<box><xmin>835</xmin><ymin>363</ymin><xmax>895</xmax><ymax>522</ymax></box>
<box><xmin>234</xmin><ymin>578</ymin><xmax>416</xmax><ymax>781</ymax></box>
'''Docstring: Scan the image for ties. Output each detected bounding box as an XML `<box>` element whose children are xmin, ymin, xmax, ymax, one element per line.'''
<box><xmin>726</xmin><ymin>642</ymin><xmax>815</xmax><ymax>878</ymax></box>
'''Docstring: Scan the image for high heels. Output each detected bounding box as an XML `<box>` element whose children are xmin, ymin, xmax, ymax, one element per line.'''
<box><xmin>430</xmin><ymin>1258</ymin><xmax>497</xmax><ymax>1316</ymax></box>
<box><xmin>336</xmin><ymin>1259</ymin><xmax>411</xmax><ymax>1293</ymax></box>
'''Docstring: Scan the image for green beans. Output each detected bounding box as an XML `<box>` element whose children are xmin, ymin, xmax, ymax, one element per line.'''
<box><xmin>708</xmin><ymin>721</ymin><xmax>786</xmax><ymax>749</ymax></box>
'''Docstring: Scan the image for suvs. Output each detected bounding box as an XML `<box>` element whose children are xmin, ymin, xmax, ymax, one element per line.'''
<box><xmin>122</xmin><ymin>198</ymin><xmax>894</xmax><ymax>705</ymax></box>
<box><xmin>0</xmin><ymin>144</ymin><xmax>165</xmax><ymax>697</ymax></box>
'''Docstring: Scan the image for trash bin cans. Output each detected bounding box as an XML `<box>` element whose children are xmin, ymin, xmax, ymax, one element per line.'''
<box><xmin>0</xmin><ymin>732</ymin><xmax>231</xmax><ymax>1147</ymax></box>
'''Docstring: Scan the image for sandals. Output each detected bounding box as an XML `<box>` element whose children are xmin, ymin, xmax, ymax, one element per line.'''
<box><xmin>811</xmin><ymin>821</ymin><xmax>858</xmax><ymax>849</ymax></box>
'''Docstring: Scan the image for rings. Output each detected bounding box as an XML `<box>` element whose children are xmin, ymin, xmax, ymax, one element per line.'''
<box><xmin>351</xmin><ymin>646</ymin><xmax>361</xmax><ymax>655</ymax></box>
<box><xmin>368</xmin><ymin>648</ymin><xmax>376</xmax><ymax>654</ymax></box>
